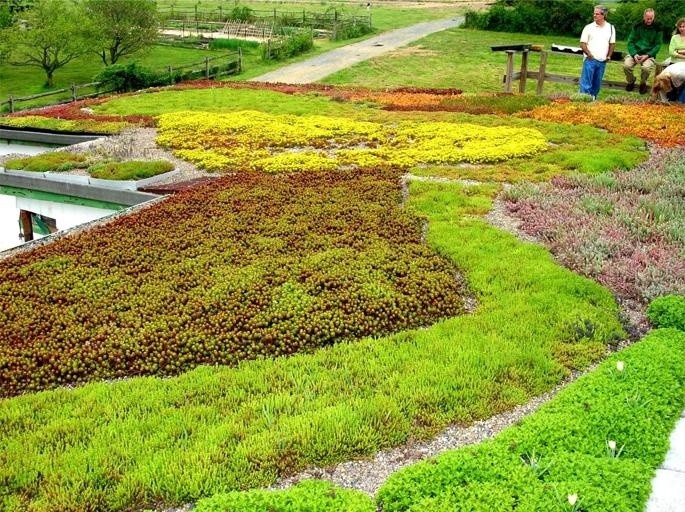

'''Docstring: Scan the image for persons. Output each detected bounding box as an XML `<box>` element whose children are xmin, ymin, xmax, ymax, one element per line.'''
<box><xmin>622</xmin><ymin>8</ymin><xmax>662</xmax><ymax>96</ymax></box>
<box><xmin>668</xmin><ymin>17</ymin><xmax>685</xmax><ymax>66</ymax></box>
<box><xmin>577</xmin><ymin>3</ymin><xmax>617</xmax><ymax>100</ymax></box>
<box><xmin>649</xmin><ymin>61</ymin><xmax>685</xmax><ymax>104</ymax></box>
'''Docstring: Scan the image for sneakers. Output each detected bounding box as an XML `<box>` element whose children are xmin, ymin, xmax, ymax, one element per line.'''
<box><xmin>639</xmin><ymin>81</ymin><xmax>647</xmax><ymax>94</ymax></box>
<box><xmin>625</xmin><ymin>76</ymin><xmax>636</xmax><ymax>91</ymax></box>
